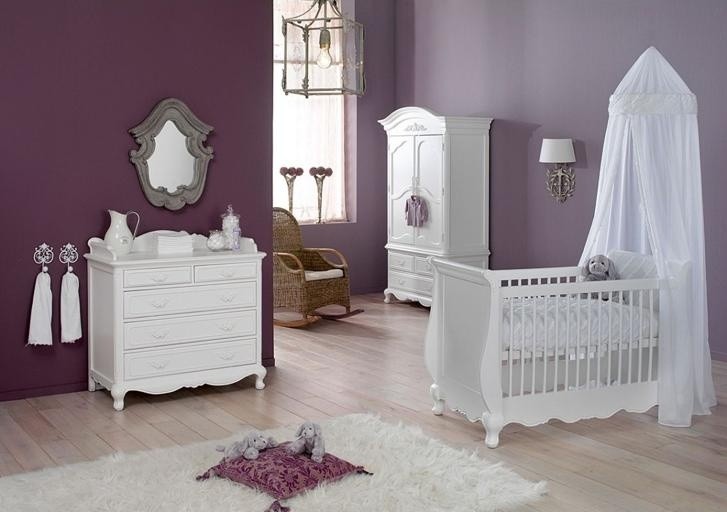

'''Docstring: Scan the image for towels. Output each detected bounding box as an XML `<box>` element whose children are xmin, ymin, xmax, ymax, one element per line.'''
<box><xmin>26</xmin><ymin>271</ymin><xmax>82</xmax><ymax>347</ymax></box>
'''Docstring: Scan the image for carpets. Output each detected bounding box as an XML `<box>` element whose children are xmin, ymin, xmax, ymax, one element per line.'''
<box><xmin>1</xmin><ymin>411</ymin><xmax>550</xmax><ymax>511</ymax></box>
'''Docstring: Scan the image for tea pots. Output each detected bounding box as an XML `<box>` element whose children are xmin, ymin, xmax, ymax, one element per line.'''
<box><xmin>104</xmin><ymin>209</ymin><xmax>140</xmax><ymax>258</ymax></box>
<box><xmin>207</xmin><ymin>229</ymin><xmax>230</xmax><ymax>251</ymax></box>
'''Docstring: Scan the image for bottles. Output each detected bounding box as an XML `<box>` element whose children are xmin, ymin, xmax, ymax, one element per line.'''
<box><xmin>220</xmin><ymin>204</ymin><xmax>241</xmax><ymax>249</ymax></box>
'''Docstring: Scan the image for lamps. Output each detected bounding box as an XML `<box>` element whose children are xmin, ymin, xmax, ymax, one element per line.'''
<box><xmin>539</xmin><ymin>138</ymin><xmax>577</xmax><ymax>205</ymax></box>
<box><xmin>281</xmin><ymin>0</ymin><xmax>365</xmax><ymax>99</ymax></box>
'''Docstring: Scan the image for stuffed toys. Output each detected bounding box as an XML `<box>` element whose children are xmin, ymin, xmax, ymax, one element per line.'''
<box><xmin>286</xmin><ymin>422</ymin><xmax>325</xmax><ymax>464</ymax></box>
<box><xmin>580</xmin><ymin>255</ymin><xmax>619</xmax><ymax>303</ymax></box>
<box><xmin>216</xmin><ymin>431</ymin><xmax>278</xmax><ymax>463</ymax></box>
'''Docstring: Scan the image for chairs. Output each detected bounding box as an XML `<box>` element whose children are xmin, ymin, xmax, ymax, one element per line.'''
<box><xmin>274</xmin><ymin>207</ymin><xmax>364</xmax><ymax>327</ymax></box>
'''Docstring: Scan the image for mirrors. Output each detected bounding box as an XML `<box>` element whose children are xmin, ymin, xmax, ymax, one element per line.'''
<box><xmin>129</xmin><ymin>98</ymin><xmax>218</xmax><ymax>211</ymax></box>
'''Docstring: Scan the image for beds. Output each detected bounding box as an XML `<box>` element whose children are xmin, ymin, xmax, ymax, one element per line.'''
<box><xmin>425</xmin><ymin>247</ymin><xmax>695</xmax><ymax>445</ymax></box>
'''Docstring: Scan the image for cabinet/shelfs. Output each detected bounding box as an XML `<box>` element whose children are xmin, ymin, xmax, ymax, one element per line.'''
<box><xmin>82</xmin><ymin>229</ymin><xmax>266</xmax><ymax>413</ymax></box>
<box><xmin>377</xmin><ymin>106</ymin><xmax>493</xmax><ymax>310</ymax></box>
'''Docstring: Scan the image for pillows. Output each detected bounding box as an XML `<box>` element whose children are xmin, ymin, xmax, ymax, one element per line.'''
<box><xmin>195</xmin><ymin>445</ymin><xmax>373</xmax><ymax>511</ymax></box>
